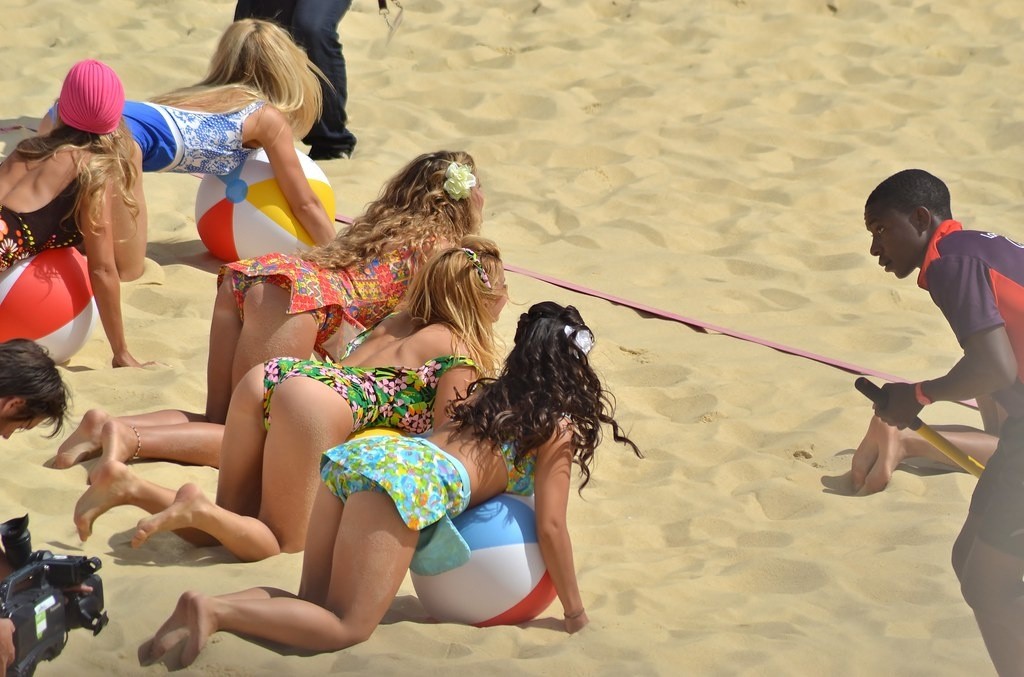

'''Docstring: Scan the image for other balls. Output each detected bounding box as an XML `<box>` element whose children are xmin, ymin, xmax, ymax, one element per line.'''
<box><xmin>193</xmin><ymin>144</ymin><xmax>336</xmax><ymax>261</ymax></box>
<box><xmin>408</xmin><ymin>494</ymin><xmax>558</xmax><ymax>627</ymax></box>
<box><xmin>0</xmin><ymin>245</ymin><xmax>98</xmax><ymax>365</ymax></box>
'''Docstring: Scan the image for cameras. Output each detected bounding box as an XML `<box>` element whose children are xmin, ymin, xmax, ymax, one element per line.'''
<box><xmin>0</xmin><ymin>513</ymin><xmax>53</xmax><ymax>570</ymax></box>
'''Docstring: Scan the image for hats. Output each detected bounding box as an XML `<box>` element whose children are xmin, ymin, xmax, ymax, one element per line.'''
<box><xmin>58</xmin><ymin>60</ymin><xmax>125</xmax><ymax>135</ymax></box>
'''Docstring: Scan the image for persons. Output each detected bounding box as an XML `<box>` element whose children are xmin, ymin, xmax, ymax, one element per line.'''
<box><xmin>0</xmin><ymin>57</ymin><xmax>156</xmax><ymax>370</ymax></box>
<box><xmin>38</xmin><ymin>18</ymin><xmax>337</xmax><ymax>248</ymax></box>
<box><xmin>864</xmin><ymin>169</ymin><xmax>1023</xmax><ymax>676</ymax></box>
<box><xmin>49</xmin><ymin>149</ymin><xmax>486</xmax><ymax>484</ymax></box>
<box><xmin>0</xmin><ymin>338</ymin><xmax>70</xmax><ymax>441</ymax></box>
<box><xmin>233</xmin><ymin>0</ymin><xmax>359</xmax><ymax>161</ymax></box>
<box><xmin>852</xmin><ymin>416</ymin><xmax>1000</xmax><ymax>496</ymax></box>
<box><xmin>150</xmin><ymin>298</ymin><xmax>643</xmax><ymax>666</ymax></box>
<box><xmin>73</xmin><ymin>233</ymin><xmax>508</xmax><ymax>563</ymax></box>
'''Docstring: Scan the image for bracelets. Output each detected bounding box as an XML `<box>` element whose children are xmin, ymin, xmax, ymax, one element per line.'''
<box><xmin>564</xmin><ymin>606</ymin><xmax>585</xmax><ymax>620</ymax></box>
<box><xmin>915</xmin><ymin>381</ymin><xmax>934</xmax><ymax>405</ymax></box>
